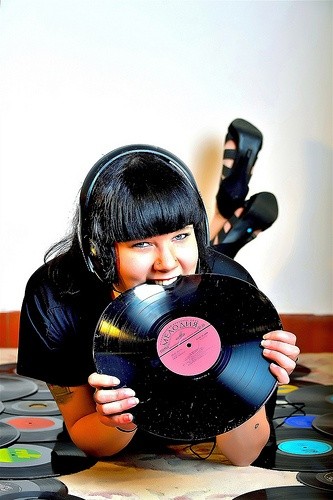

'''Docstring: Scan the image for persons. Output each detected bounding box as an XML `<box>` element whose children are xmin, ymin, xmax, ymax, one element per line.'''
<box><xmin>17</xmin><ymin>118</ymin><xmax>300</xmax><ymax>466</ymax></box>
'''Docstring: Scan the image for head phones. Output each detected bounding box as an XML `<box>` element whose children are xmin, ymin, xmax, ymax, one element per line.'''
<box><xmin>75</xmin><ymin>144</ymin><xmax>210</xmax><ymax>284</ymax></box>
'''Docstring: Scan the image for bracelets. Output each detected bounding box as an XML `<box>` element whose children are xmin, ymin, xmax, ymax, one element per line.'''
<box><xmin>116</xmin><ymin>426</ymin><xmax>138</xmax><ymax>432</ymax></box>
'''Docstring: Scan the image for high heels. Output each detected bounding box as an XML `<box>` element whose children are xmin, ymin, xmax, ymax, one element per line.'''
<box><xmin>209</xmin><ymin>192</ymin><xmax>279</xmax><ymax>259</ymax></box>
<box><xmin>215</xmin><ymin>119</ymin><xmax>263</xmax><ymax>219</ymax></box>
